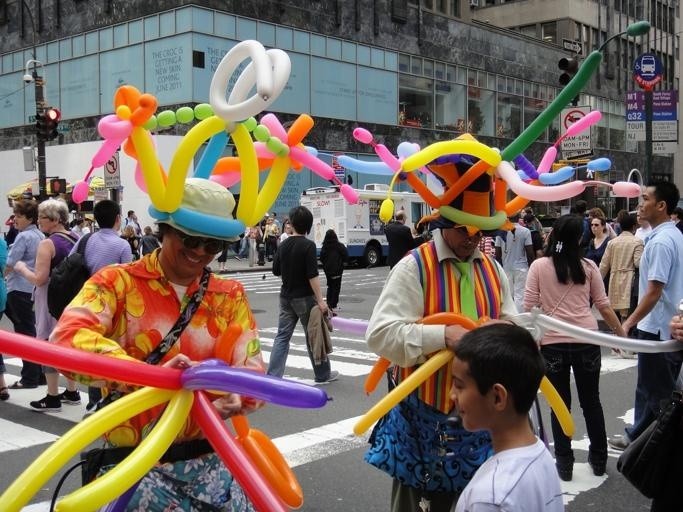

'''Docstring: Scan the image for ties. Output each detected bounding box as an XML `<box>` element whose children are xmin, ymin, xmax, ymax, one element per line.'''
<box><xmin>454</xmin><ymin>260</ymin><xmax>478</xmax><ymax>322</ymax></box>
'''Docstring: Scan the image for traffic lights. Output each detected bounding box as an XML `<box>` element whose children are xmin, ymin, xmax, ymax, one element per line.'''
<box><xmin>48</xmin><ymin>106</ymin><xmax>60</xmax><ymax>141</ymax></box>
<box><xmin>50</xmin><ymin>179</ymin><xmax>66</xmax><ymax>193</ymax></box>
<box><xmin>35</xmin><ymin>112</ymin><xmax>47</xmax><ymax>141</ymax></box>
<box><xmin>558</xmin><ymin>57</ymin><xmax>580</xmax><ymax>102</ymax></box>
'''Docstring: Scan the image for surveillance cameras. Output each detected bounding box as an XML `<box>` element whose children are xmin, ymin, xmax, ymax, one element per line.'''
<box><xmin>23</xmin><ymin>75</ymin><xmax>33</xmax><ymax>84</ymax></box>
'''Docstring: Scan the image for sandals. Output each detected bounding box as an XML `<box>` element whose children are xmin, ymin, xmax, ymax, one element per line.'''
<box><xmin>9</xmin><ymin>381</ymin><xmax>37</xmax><ymax>389</ymax></box>
<box><xmin>0</xmin><ymin>387</ymin><xmax>9</xmax><ymax>399</ymax></box>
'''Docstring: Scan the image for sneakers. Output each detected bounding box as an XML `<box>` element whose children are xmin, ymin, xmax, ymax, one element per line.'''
<box><xmin>556</xmin><ymin>463</ymin><xmax>573</xmax><ymax>481</ymax></box>
<box><xmin>587</xmin><ymin>455</ymin><xmax>606</xmax><ymax>476</ymax></box>
<box><xmin>58</xmin><ymin>390</ymin><xmax>82</xmax><ymax>405</ymax></box>
<box><xmin>609</xmin><ymin>437</ymin><xmax>629</xmax><ymax>451</ymax></box>
<box><xmin>30</xmin><ymin>394</ymin><xmax>62</xmax><ymax>411</ymax></box>
<box><xmin>315</xmin><ymin>371</ymin><xmax>339</xmax><ymax>383</ymax></box>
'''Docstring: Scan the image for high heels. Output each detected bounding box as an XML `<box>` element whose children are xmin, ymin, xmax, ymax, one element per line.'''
<box><xmin>612</xmin><ymin>347</ymin><xmax>635</xmax><ymax>359</ymax></box>
<box><xmin>331</xmin><ymin>310</ymin><xmax>337</xmax><ymax>316</ymax></box>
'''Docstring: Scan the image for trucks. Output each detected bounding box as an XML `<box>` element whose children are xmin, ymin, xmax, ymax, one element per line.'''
<box><xmin>299</xmin><ymin>183</ymin><xmax>433</xmax><ymax>266</ymax></box>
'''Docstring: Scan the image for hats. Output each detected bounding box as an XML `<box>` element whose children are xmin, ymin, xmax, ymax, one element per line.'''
<box><xmin>415</xmin><ymin>134</ymin><xmax>515</xmax><ymax>239</ymax></box>
<box><xmin>523</xmin><ymin>212</ymin><xmax>539</xmax><ymax>224</ymax></box>
<box><xmin>154</xmin><ymin>178</ymin><xmax>240</xmax><ymax>242</ymax></box>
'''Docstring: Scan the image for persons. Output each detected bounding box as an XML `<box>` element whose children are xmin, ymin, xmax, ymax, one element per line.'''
<box><xmin>123</xmin><ymin>211</ymin><xmax>141</xmax><ymax>237</ymax></box>
<box><xmin>271</xmin><ymin>212</ymin><xmax>280</xmax><ymax>228</ymax></box>
<box><xmin>589</xmin><ymin>207</ymin><xmax>617</xmax><ymax>239</ymax></box>
<box><xmin>268</xmin><ymin>206</ymin><xmax>339</xmax><ymax>382</ymax></box>
<box><xmin>119</xmin><ymin>225</ymin><xmax>143</xmax><ymax>260</ymax></box>
<box><xmin>47</xmin><ymin>199</ymin><xmax>133</xmax><ymax>412</ymax></box>
<box><xmin>385</xmin><ymin>210</ymin><xmax>424</xmax><ymax>270</ymax></box>
<box><xmin>71</xmin><ymin>218</ymin><xmax>84</xmax><ymax>237</ymax></box>
<box><xmin>234</xmin><ymin>226</ymin><xmax>250</xmax><ymax>261</ymax></box>
<box><xmin>583</xmin><ymin>218</ymin><xmax>611</xmax><ymax>267</ymax></box>
<box><xmin>261</xmin><ymin>213</ymin><xmax>270</xmax><ymax>235</ymax></box>
<box><xmin>522</xmin><ymin>214</ymin><xmax>626</xmax><ymax>482</ymax></box>
<box><xmin>217</xmin><ymin>244</ymin><xmax>229</xmax><ymax>271</ymax></box>
<box><xmin>521</xmin><ymin>206</ymin><xmax>546</xmax><ymax>241</ymax></box>
<box><xmin>0</xmin><ymin>238</ymin><xmax>9</xmax><ymax>400</ymax></box>
<box><xmin>320</xmin><ymin>230</ymin><xmax>349</xmax><ymax>313</ymax></box>
<box><xmin>361</xmin><ymin>156</ymin><xmax>540</xmax><ymax>511</ymax></box>
<box><xmin>263</xmin><ymin>217</ymin><xmax>280</xmax><ymax>262</ymax></box>
<box><xmin>14</xmin><ymin>197</ymin><xmax>82</xmax><ymax>412</ymax></box>
<box><xmin>5</xmin><ymin>215</ymin><xmax>18</xmax><ymax>243</ymax></box>
<box><xmin>596</xmin><ymin>209</ymin><xmax>645</xmax><ymax>323</ymax></box>
<box><xmin>671</xmin><ymin>207</ymin><xmax>682</xmax><ymax>232</ymax></box>
<box><xmin>137</xmin><ymin>226</ymin><xmax>160</xmax><ymax>256</ymax></box>
<box><xmin>608</xmin><ymin>180</ymin><xmax>683</xmax><ymax>451</ymax></box>
<box><xmin>495</xmin><ymin>214</ymin><xmax>535</xmax><ymax>313</ymax></box>
<box><xmin>524</xmin><ymin>215</ymin><xmax>542</xmax><ymax>259</ymax></box>
<box><xmin>49</xmin><ymin>177</ymin><xmax>268</xmax><ymax>512</ymax></box>
<box><xmin>449</xmin><ymin>324</ymin><xmax>565</xmax><ymax>511</ymax></box>
<box><xmin>279</xmin><ymin>223</ymin><xmax>293</xmax><ymax>243</ymax></box>
<box><xmin>635</xmin><ymin>206</ymin><xmax>653</xmax><ymax>243</ymax></box>
<box><xmin>617</xmin><ymin>314</ymin><xmax>683</xmax><ymax>512</ymax></box>
<box><xmin>574</xmin><ymin>200</ymin><xmax>591</xmax><ymax>243</ymax></box>
<box><xmin>3</xmin><ymin>199</ymin><xmax>47</xmax><ymax>389</ymax></box>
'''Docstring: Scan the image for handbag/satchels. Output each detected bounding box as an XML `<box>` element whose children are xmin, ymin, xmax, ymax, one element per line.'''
<box><xmin>632</xmin><ymin>245</ymin><xmax>644</xmax><ymax>302</ymax></box>
<box><xmin>617</xmin><ymin>365</ymin><xmax>682</xmax><ymax>499</ymax></box>
<box><xmin>362</xmin><ymin>368</ymin><xmax>494</xmax><ymax>493</ymax></box>
<box><xmin>50</xmin><ymin>439</ymin><xmax>256</xmax><ymax>512</ymax></box>
<box><xmin>80</xmin><ymin>266</ymin><xmax>211</xmax><ymax>455</ymax></box>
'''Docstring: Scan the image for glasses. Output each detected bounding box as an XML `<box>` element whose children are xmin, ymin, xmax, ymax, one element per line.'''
<box><xmin>38</xmin><ymin>216</ymin><xmax>50</xmax><ymax>220</ymax></box>
<box><xmin>591</xmin><ymin>223</ymin><xmax>602</xmax><ymax>226</ymax></box>
<box><xmin>170</xmin><ymin>227</ymin><xmax>225</xmax><ymax>255</ymax></box>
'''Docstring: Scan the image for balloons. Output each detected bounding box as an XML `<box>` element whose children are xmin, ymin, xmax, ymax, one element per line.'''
<box><xmin>68</xmin><ymin>39</ymin><xmax>358</xmax><ymax>236</ymax></box>
<box><xmin>305</xmin><ymin>16</ymin><xmax>649</xmax><ymax>229</ymax></box>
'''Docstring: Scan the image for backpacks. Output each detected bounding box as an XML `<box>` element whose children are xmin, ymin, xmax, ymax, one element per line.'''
<box><xmin>47</xmin><ymin>233</ymin><xmax>94</xmax><ymax>321</ymax></box>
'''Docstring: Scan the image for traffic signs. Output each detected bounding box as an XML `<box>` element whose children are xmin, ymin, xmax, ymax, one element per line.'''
<box><xmin>633</xmin><ymin>53</ymin><xmax>664</xmax><ymax>88</ymax></box>
<box><xmin>104</xmin><ymin>152</ymin><xmax>120</xmax><ymax>188</ymax></box>
<box><xmin>560</xmin><ymin>106</ymin><xmax>591</xmax><ymax>150</ymax></box>
